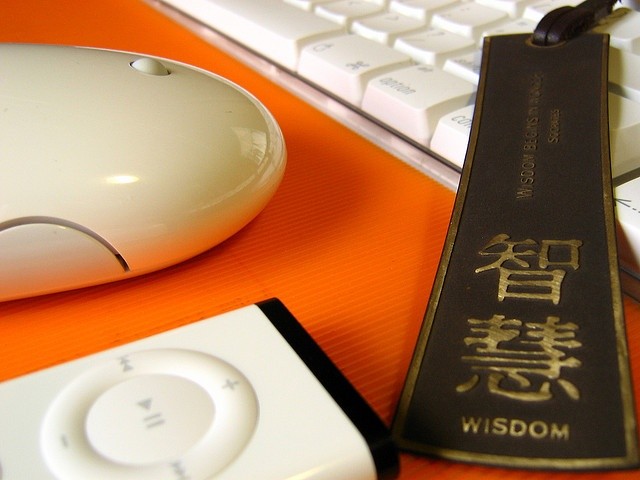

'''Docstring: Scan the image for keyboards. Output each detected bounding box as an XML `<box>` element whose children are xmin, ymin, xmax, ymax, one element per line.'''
<box><xmin>144</xmin><ymin>1</ymin><xmax>639</xmax><ymax>305</ymax></box>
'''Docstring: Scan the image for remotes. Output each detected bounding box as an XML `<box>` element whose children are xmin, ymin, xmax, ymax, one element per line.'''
<box><xmin>1</xmin><ymin>298</ymin><xmax>399</xmax><ymax>479</ymax></box>
